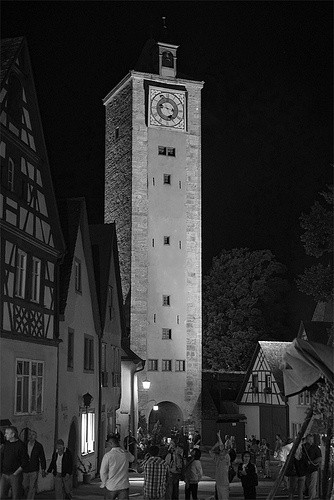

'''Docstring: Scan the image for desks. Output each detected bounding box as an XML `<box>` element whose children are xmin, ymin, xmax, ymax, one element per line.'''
<box><xmin>265</xmin><ymin>460</ymin><xmax>282</xmax><ymax>479</ymax></box>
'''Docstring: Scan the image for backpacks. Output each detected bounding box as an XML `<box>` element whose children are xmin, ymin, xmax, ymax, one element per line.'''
<box><xmin>228</xmin><ymin>466</ymin><xmax>236</xmax><ymax>483</ymax></box>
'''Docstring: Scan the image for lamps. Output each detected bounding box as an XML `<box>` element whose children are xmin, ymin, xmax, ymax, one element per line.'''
<box><xmin>138</xmin><ymin>399</ymin><xmax>158</xmax><ymax>411</ymax></box>
<box><xmin>137</xmin><ymin>377</ymin><xmax>151</xmax><ymax>392</ymax></box>
<box><xmin>79</xmin><ymin>392</ymin><xmax>93</xmax><ymax>417</ymax></box>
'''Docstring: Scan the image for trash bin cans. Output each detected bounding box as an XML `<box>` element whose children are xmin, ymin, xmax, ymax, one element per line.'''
<box><xmin>216</xmin><ymin>414</ymin><xmax>247</xmax><ymax>453</ymax></box>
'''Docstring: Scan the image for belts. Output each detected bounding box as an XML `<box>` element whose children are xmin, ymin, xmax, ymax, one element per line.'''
<box><xmin>2</xmin><ymin>472</ymin><xmax>13</xmax><ymax>474</ymax></box>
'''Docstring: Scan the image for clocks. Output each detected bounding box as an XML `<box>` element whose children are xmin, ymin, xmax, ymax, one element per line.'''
<box><xmin>148</xmin><ymin>85</ymin><xmax>186</xmax><ymax>133</ymax></box>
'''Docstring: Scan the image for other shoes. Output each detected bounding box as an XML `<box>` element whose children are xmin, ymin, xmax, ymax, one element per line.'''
<box><xmin>304</xmin><ymin>496</ymin><xmax>309</xmax><ymax>498</ymax></box>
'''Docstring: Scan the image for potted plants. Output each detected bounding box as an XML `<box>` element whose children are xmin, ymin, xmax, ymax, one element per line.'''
<box><xmin>76</xmin><ymin>456</ymin><xmax>92</xmax><ymax>484</ymax></box>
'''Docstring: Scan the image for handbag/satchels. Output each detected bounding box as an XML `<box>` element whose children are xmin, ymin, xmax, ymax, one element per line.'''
<box><xmin>181</xmin><ymin>475</ymin><xmax>185</xmax><ymax>481</ymax></box>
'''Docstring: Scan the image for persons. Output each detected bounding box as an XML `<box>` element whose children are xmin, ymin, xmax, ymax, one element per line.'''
<box><xmin>237</xmin><ymin>451</ymin><xmax>258</xmax><ymax>500</ymax></box>
<box><xmin>99</xmin><ymin>436</ymin><xmax>135</xmax><ymax>500</ymax></box>
<box><xmin>209</xmin><ymin>430</ymin><xmax>237</xmax><ymax>500</ymax></box>
<box><xmin>0</xmin><ymin>427</ymin><xmax>29</xmax><ymax>500</ymax></box>
<box><xmin>224</xmin><ymin>434</ymin><xmax>282</xmax><ymax>478</ymax></box>
<box><xmin>137</xmin><ymin>446</ymin><xmax>170</xmax><ymax>500</ymax></box>
<box><xmin>45</xmin><ymin>439</ymin><xmax>74</xmax><ymax>500</ymax></box>
<box><xmin>279</xmin><ymin>433</ymin><xmax>334</xmax><ymax>500</ymax></box>
<box><xmin>183</xmin><ymin>448</ymin><xmax>202</xmax><ymax>500</ymax></box>
<box><xmin>22</xmin><ymin>430</ymin><xmax>47</xmax><ymax>500</ymax></box>
<box><xmin>124</xmin><ymin>430</ymin><xmax>201</xmax><ymax>500</ymax></box>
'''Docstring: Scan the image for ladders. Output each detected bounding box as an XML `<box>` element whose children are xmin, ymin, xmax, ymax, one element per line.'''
<box><xmin>267</xmin><ymin>410</ymin><xmax>313</xmax><ymax>500</ymax></box>
